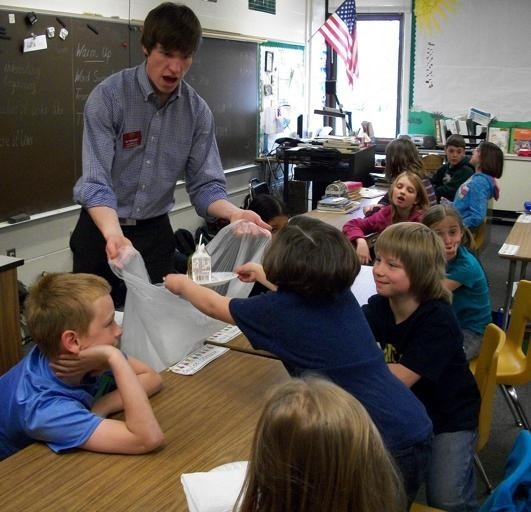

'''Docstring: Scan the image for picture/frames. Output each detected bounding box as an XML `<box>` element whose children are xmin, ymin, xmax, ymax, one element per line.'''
<box><xmin>265</xmin><ymin>50</ymin><xmax>273</xmax><ymax>72</ymax></box>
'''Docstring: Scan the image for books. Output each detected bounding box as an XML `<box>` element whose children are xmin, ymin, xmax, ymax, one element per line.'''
<box><xmin>436</xmin><ymin>118</ymin><xmax>531</xmax><ymax>154</ymax></box>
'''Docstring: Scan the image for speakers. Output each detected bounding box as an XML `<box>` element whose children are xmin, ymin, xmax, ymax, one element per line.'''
<box><xmin>287</xmin><ymin>181</ymin><xmax>308</xmax><ymax>212</ymax></box>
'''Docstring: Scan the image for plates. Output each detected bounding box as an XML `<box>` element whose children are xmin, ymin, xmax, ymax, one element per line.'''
<box><xmin>187</xmin><ymin>272</ymin><xmax>238</xmax><ymax>287</ymax></box>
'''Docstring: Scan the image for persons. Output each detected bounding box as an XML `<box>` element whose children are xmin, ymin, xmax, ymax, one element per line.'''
<box><xmin>68</xmin><ymin>2</ymin><xmax>274</xmax><ymax>314</ymax></box>
<box><xmin>0</xmin><ymin>272</ymin><xmax>165</xmax><ymax>461</ymax></box>
<box><xmin>163</xmin><ymin>133</ymin><xmax>504</xmax><ymax>512</ymax></box>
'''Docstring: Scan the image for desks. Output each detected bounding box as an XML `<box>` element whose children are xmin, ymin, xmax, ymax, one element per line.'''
<box><xmin>0</xmin><ymin>341</ymin><xmax>292</xmax><ymax>512</ymax></box>
<box><xmin>278</xmin><ymin>136</ymin><xmax>377</xmax><ymax>211</ymax></box>
<box><xmin>291</xmin><ymin>184</ymin><xmax>391</xmax><ymax>250</ymax></box>
<box><xmin>0</xmin><ymin>254</ymin><xmax>24</xmax><ymax>378</ymax></box>
<box><xmin>203</xmin><ymin>323</ymin><xmax>281</xmax><ymax>359</ymax></box>
<box><xmin>497</xmin><ymin>212</ymin><xmax>531</xmax><ymax>332</ymax></box>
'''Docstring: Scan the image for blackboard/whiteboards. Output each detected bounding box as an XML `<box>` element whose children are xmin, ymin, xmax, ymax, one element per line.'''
<box><xmin>0</xmin><ymin>6</ymin><xmax>261</xmax><ymax>229</ymax></box>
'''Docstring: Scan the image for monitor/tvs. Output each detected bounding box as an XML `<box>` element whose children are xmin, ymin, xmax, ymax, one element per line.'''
<box><xmin>297</xmin><ymin>114</ymin><xmax>303</xmax><ymax>138</ymax></box>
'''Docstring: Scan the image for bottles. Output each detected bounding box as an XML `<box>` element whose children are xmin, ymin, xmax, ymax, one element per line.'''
<box><xmin>192</xmin><ymin>246</ymin><xmax>211</xmax><ymax>283</ymax></box>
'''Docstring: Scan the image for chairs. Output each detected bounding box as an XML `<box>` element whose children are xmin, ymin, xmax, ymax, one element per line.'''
<box><xmin>410</xmin><ymin>429</ymin><xmax>531</xmax><ymax>512</ymax></box>
<box><xmin>472</xmin><ymin>323</ymin><xmax>506</xmax><ymax>493</ymax></box>
<box><xmin>469</xmin><ymin>221</ymin><xmax>485</xmax><ymax>251</ymax></box>
<box><xmin>467</xmin><ymin>280</ymin><xmax>531</xmax><ymax>432</ymax></box>
<box><xmin>421</xmin><ymin>154</ymin><xmax>444</xmax><ymax>179</ymax></box>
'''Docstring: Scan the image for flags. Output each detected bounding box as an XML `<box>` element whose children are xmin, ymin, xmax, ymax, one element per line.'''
<box><xmin>317</xmin><ymin>0</ymin><xmax>361</xmax><ymax>89</ymax></box>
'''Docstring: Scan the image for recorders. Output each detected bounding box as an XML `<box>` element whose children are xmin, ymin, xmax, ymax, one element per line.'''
<box><xmin>398</xmin><ymin>135</ymin><xmax>434</xmax><ymax>148</ymax></box>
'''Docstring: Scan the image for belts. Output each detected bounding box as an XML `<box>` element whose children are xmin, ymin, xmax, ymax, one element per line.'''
<box><xmin>118</xmin><ymin>217</ymin><xmax>136</xmax><ymax>226</ymax></box>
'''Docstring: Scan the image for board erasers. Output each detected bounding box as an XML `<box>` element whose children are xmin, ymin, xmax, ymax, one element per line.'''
<box><xmin>8</xmin><ymin>212</ymin><xmax>30</xmax><ymax>223</ymax></box>
